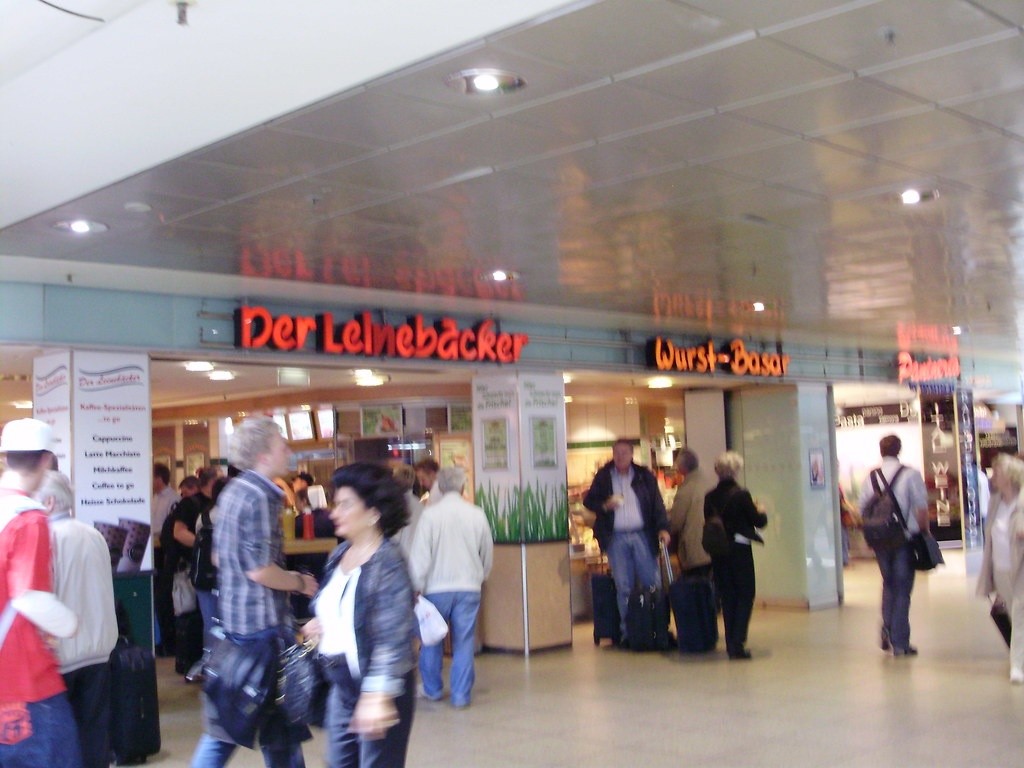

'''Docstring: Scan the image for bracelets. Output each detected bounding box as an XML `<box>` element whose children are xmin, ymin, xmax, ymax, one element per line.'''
<box><xmin>296</xmin><ymin>575</ymin><xmax>305</xmax><ymax>591</ymax></box>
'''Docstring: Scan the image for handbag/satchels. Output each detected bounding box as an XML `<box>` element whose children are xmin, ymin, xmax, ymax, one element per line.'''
<box><xmin>186</xmin><ymin>630</ymin><xmax>282</xmax><ymax>720</ymax></box>
<box><xmin>627</xmin><ymin>588</ymin><xmax>671</xmax><ymax>651</ymax></box>
<box><xmin>413</xmin><ymin>594</ymin><xmax>448</xmax><ymax>647</ymax></box>
<box><xmin>275</xmin><ymin>643</ymin><xmax>318</xmax><ymax>725</ymax></box>
<box><xmin>172</xmin><ymin>568</ymin><xmax>196</xmax><ymax>616</ymax></box>
<box><xmin>906</xmin><ymin>529</ymin><xmax>942</xmax><ymax>570</ymax></box>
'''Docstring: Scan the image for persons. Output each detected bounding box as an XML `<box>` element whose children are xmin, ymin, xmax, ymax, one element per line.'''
<box><xmin>153</xmin><ymin>464</ymin><xmax>182</xmax><ymax>657</ymax></box>
<box><xmin>195</xmin><ymin>477</ymin><xmax>229</xmax><ymax>655</ymax></box>
<box><xmin>839</xmin><ymin>487</ymin><xmax>850</xmax><ymax>567</ymax></box>
<box><xmin>669</xmin><ymin>448</ymin><xmax>712</xmax><ymax>579</ymax></box>
<box><xmin>858</xmin><ymin>435</ymin><xmax>928</xmax><ymax>657</ymax></box>
<box><xmin>190</xmin><ymin>417</ymin><xmax>319</xmax><ymax>768</ymax></box>
<box><xmin>583</xmin><ymin>440</ymin><xmax>671</xmax><ymax>650</ymax></box>
<box><xmin>28</xmin><ymin>470</ymin><xmax>119</xmax><ymax>768</ymax></box>
<box><xmin>178</xmin><ymin>475</ymin><xmax>198</xmax><ymax>498</ymax></box>
<box><xmin>287</xmin><ymin>488</ymin><xmax>344</xmax><ymax>627</ymax></box>
<box><xmin>975</xmin><ymin>454</ymin><xmax>1024</xmax><ymax>684</ymax></box>
<box><xmin>703</xmin><ymin>450</ymin><xmax>767</xmax><ymax>660</ymax></box>
<box><xmin>297</xmin><ymin>462</ymin><xmax>422</xmax><ymax>768</ymax></box>
<box><xmin>171</xmin><ymin>466</ymin><xmax>226</xmax><ymax>676</ymax></box>
<box><xmin>0</xmin><ymin>418</ymin><xmax>78</xmax><ymax>768</ymax></box>
<box><xmin>389</xmin><ymin>464</ymin><xmax>425</xmax><ymax>665</ymax></box>
<box><xmin>408</xmin><ymin>457</ymin><xmax>494</xmax><ymax>710</ymax></box>
<box><xmin>273</xmin><ymin>471</ymin><xmax>314</xmax><ymax>516</ymax></box>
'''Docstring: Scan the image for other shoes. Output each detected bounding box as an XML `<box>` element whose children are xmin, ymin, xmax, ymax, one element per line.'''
<box><xmin>417</xmin><ymin>684</ymin><xmax>442</xmax><ymax>702</ymax></box>
<box><xmin>728</xmin><ymin>648</ymin><xmax>750</xmax><ymax>659</ymax></box>
<box><xmin>882</xmin><ymin>641</ymin><xmax>891</xmax><ymax>650</ymax></box>
<box><xmin>894</xmin><ymin>647</ymin><xmax>917</xmax><ymax>656</ymax></box>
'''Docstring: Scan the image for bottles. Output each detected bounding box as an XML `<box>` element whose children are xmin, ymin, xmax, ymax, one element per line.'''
<box><xmin>302</xmin><ymin>508</ymin><xmax>316</xmax><ymax>540</ymax></box>
<box><xmin>283</xmin><ymin>508</ymin><xmax>295</xmax><ymax>541</ymax></box>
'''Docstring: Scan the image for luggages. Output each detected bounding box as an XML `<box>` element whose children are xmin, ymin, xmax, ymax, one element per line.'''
<box><xmin>592</xmin><ymin>551</ymin><xmax>621</xmax><ymax>646</ymax></box>
<box><xmin>111</xmin><ymin>636</ymin><xmax>161</xmax><ymax>765</ymax></box>
<box><xmin>987</xmin><ymin>590</ymin><xmax>1011</xmax><ymax>650</ymax></box>
<box><xmin>661</xmin><ymin>539</ymin><xmax>718</xmax><ymax>653</ymax></box>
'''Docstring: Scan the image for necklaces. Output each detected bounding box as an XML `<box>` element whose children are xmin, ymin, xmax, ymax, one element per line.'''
<box><xmin>346</xmin><ymin>533</ymin><xmax>382</xmax><ymax>566</ymax></box>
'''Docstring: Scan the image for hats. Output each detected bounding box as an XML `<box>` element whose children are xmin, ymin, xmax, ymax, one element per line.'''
<box><xmin>0</xmin><ymin>418</ymin><xmax>56</xmax><ymax>453</ymax></box>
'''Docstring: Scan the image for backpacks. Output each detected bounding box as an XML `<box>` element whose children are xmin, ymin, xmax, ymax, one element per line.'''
<box><xmin>160</xmin><ymin>495</ymin><xmax>199</xmax><ymax>550</ymax></box>
<box><xmin>191</xmin><ymin>512</ymin><xmax>218</xmax><ymax>592</ymax></box>
<box><xmin>702</xmin><ymin>488</ymin><xmax>748</xmax><ymax>554</ymax></box>
<box><xmin>862</xmin><ymin>466</ymin><xmax>906</xmax><ymax>549</ymax></box>
